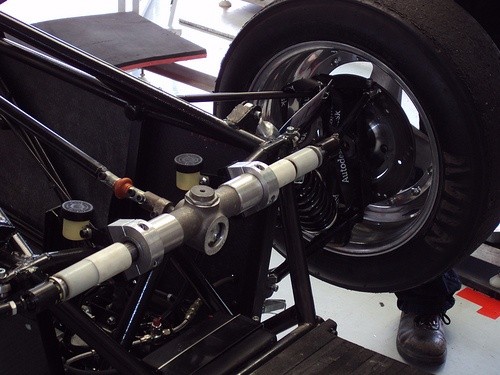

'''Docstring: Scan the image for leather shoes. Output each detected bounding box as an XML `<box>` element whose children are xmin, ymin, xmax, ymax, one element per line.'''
<box><xmin>395</xmin><ymin>308</ymin><xmax>447</xmax><ymax>365</ymax></box>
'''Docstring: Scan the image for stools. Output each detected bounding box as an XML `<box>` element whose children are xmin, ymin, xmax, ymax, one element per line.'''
<box><xmin>30</xmin><ymin>10</ymin><xmax>207</xmax><ymax>72</ymax></box>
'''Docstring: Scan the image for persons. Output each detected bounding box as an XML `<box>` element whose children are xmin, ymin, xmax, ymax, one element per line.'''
<box><xmin>393</xmin><ymin>0</ymin><xmax>500</xmax><ymax>367</ymax></box>
